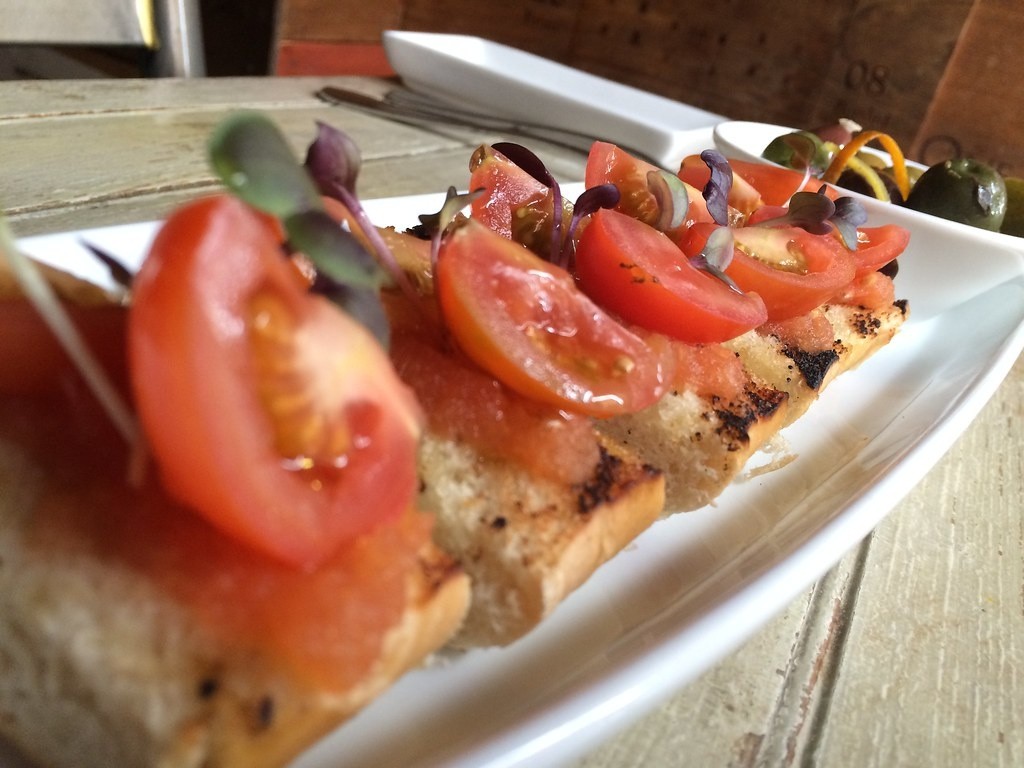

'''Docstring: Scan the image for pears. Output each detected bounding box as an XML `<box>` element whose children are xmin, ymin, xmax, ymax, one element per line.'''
<box><xmin>760</xmin><ymin>130</ymin><xmax>1024</xmax><ymax>238</ymax></box>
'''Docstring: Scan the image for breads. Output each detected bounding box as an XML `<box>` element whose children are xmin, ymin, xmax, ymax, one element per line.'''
<box><xmin>0</xmin><ymin>224</ymin><xmax>907</xmax><ymax>768</ymax></box>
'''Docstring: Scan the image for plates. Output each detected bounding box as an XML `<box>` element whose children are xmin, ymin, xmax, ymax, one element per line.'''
<box><xmin>11</xmin><ymin>180</ymin><xmax>1024</xmax><ymax>768</ymax></box>
<box><xmin>383</xmin><ymin>29</ymin><xmax>733</xmax><ymax>167</ymax></box>
<box><xmin>714</xmin><ymin>119</ymin><xmax>1024</xmax><ymax>248</ymax></box>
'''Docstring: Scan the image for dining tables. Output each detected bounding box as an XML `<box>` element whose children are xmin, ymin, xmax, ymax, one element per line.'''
<box><xmin>0</xmin><ymin>75</ymin><xmax>1024</xmax><ymax>768</ymax></box>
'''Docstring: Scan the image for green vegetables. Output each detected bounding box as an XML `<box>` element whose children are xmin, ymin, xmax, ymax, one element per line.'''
<box><xmin>205</xmin><ymin>109</ymin><xmax>866</xmax><ymax>353</ymax></box>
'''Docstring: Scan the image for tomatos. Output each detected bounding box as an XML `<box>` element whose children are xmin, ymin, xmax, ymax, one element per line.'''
<box><xmin>126</xmin><ymin>140</ymin><xmax>910</xmax><ymax>574</ymax></box>
